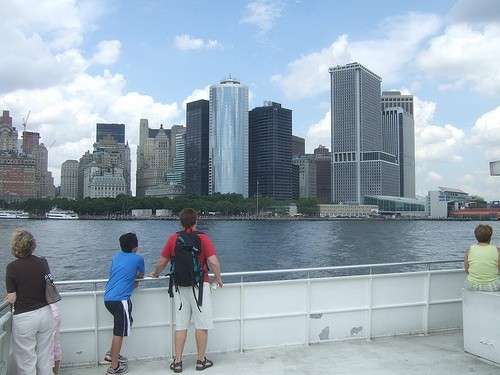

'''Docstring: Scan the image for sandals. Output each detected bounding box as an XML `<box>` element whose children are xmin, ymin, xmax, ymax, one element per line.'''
<box><xmin>170</xmin><ymin>359</ymin><xmax>182</xmax><ymax>373</ymax></box>
<box><xmin>196</xmin><ymin>356</ymin><xmax>213</xmax><ymax>371</ymax></box>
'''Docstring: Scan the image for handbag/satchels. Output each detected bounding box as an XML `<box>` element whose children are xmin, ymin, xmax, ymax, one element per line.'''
<box><xmin>44</xmin><ymin>274</ymin><xmax>61</xmax><ymax>304</ymax></box>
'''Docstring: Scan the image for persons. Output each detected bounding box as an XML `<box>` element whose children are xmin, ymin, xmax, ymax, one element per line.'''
<box><xmin>464</xmin><ymin>225</ymin><xmax>500</xmax><ymax>292</ymax></box>
<box><xmin>148</xmin><ymin>207</ymin><xmax>222</xmax><ymax>373</ymax></box>
<box><xmin>6</xmin><ymin>230</ymin><xmax>64</xmax><ymax>375</ymax></box>
<box><xmin>104</xmin><ymin>232</ymin><xmax>145</xmax><ymax>375</ymax></box>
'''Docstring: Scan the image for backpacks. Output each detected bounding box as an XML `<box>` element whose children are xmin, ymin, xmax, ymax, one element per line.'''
<box><xmin>165</xmin><ymin>227</ymin><xmax>206</xmax><ymax>312</ymax></box>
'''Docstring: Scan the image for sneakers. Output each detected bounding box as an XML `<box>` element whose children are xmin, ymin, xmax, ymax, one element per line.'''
<box><xmin>104</xmin><ymin>351</ymin><xmax>128</xmax><ymax>363</ymax></box>
<box><xmin>104</xmin><ymin>362</ymin><xmax>129</xmax><ymax>375</ymax></box>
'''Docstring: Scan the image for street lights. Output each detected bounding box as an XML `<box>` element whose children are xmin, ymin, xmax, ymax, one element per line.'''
<box><xmin>256</xmin><ymin>175</ymin><xmax>262</xmax><ymax>218</ymax></box>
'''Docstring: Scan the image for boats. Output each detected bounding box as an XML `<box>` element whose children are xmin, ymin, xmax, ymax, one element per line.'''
<box><xmin>46</xmin><ymin>203</ymin><xmax>80</xmax><ymax>220</ymax></box>
<box><xmin>0</xmin><ymin>208</ymin><xmax>30</xmax><ymax>219</ymax></box>
